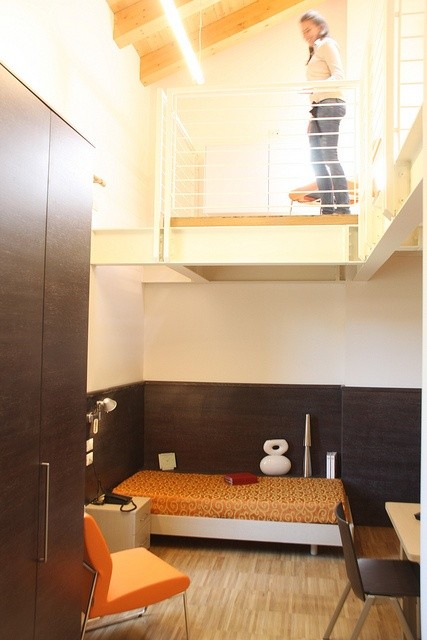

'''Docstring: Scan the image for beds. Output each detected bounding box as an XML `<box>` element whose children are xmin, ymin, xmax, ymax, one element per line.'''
<box><xmin>112</xmin><ymin>470</ymin><xmax>354</xmax><ymax>556</ymax></box>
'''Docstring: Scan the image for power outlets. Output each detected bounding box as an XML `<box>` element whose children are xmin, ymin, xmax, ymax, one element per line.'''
<box><xmin>86</xmin><ymin>452</ymin><xmax>93</xmax><ymax>467</ymax></box>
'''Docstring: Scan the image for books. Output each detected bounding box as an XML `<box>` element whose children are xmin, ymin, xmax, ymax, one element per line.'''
<box><xmin>225</xmin><ymin>472</ymin><xmax>258</xmax><ymax>485</ymax></box>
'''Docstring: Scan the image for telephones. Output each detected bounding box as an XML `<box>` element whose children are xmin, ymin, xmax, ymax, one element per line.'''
<box><xmin>104</xmin><ymin>493</ymin><xmax>137</xmax><ymax>512</ymax></box>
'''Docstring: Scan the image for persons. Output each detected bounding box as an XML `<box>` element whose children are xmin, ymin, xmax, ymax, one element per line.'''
<box><xmin>299</xmin><ymin>11</ymin><xmax>351</xmax><ymax>214</ymax></box>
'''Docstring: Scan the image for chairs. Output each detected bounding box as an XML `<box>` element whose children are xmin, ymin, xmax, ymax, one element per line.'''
<box><xmin>323</xmin><ymin>500</ymin><xmax>420</xmax><ymax>640</ymax></box>
<box><xmin>287</xmin><ymin>139</ymin><xmax>381</xmax><ymax>214</ymax></box>
<box><xmin>83</xmin><ymin>511</ymin><xmax>191</xmax><ymax>640</ymax></box>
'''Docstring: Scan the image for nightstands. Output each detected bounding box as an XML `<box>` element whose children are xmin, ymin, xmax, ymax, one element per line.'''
<box><xmin>85</xmin><ymin>494</ymin><xmax>152</xmax><ymax>550</ymax></box>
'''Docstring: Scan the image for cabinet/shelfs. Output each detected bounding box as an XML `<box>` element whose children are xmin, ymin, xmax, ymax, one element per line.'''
<box><xmin>0</xmin><ymin>60</ymin><xmax>95</xmax><ymax>639</ymax></box>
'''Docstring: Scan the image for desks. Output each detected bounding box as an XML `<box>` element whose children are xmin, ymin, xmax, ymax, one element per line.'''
<box><xmin>385</xmin><ymin>502</ymin><xmax>420</xmax><ymax>640</ymax></box>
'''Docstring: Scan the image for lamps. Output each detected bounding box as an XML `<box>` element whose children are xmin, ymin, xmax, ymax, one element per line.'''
<box><xmin>86</xmin><ymin>398</ymin><xmax>117</xmax><ymax>424</ymax></box>
